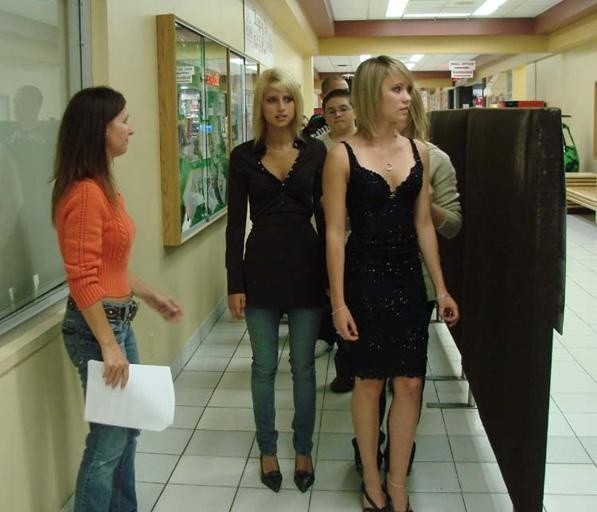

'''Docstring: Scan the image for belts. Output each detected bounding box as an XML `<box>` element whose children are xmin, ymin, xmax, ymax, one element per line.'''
<box><xmin>66</xmin><ymin>294</ymin><xmax>138</xmax><ymax>320</ymax></box>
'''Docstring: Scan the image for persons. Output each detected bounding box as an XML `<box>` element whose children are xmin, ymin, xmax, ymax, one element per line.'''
<box><xmin>225</xmin><ymin>69</ymin><xmax>329</xmax><ymax>493</ymax></box>
<box><xmin>302</xmin><ymin>76</ymin><xmax>360</xmax><ymax>392</ymax></box>
<box><xmin>336</xmin><ymin>91</ymin><xmax>464</xmax><ymax>471</ymax></box>
<box><xmin>0</xmin><ymin>86</ymin><xmax>60</xmax><ymax>311</ymax></box>
<box><xmin>323</xmin><ymin>56</ymin><xmax>460</xmax><ymax>512</ymax></box>
<box><xmin>48</xmin><ymin>87</ymin><xmax>184</xmax><ymax>512</ymax></box>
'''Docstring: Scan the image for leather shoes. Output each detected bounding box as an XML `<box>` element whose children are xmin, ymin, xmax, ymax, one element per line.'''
<box><xmin>294</xmin><ymin>452</ymin><xmax>314</xmax><ymax>492</ymax></box>
<box><xmin>258</xmin><ymin>453</ymin><xmax>281</xmax><ymax>491</ymax></box>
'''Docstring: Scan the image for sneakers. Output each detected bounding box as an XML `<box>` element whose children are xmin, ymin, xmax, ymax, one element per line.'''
<box><xmin>353</xmin><ymin>442</ymin><xmax>415</xmax><ymax>476</ymax></box>
<box><xmin>314</xmin><ymin>339</ymin><xmax>334</xmax><ymax>357</ymax></box>
<box><xmin>331</xmin><ymin>372</ymin><xmax>356</xmax><ymax>392</ymax></box>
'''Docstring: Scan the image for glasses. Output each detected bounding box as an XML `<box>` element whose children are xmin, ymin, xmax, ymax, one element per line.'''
<box><xmin>324</xmin><ymin>107</ymin><xmax>353</xmax><ymax>117</ymax></box>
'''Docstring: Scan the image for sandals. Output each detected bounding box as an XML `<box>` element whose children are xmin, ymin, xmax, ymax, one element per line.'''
<box><xmin>360</xmin><ymin>480</ymin><xmax>413</xmax><ymax>512</ymax></box>
